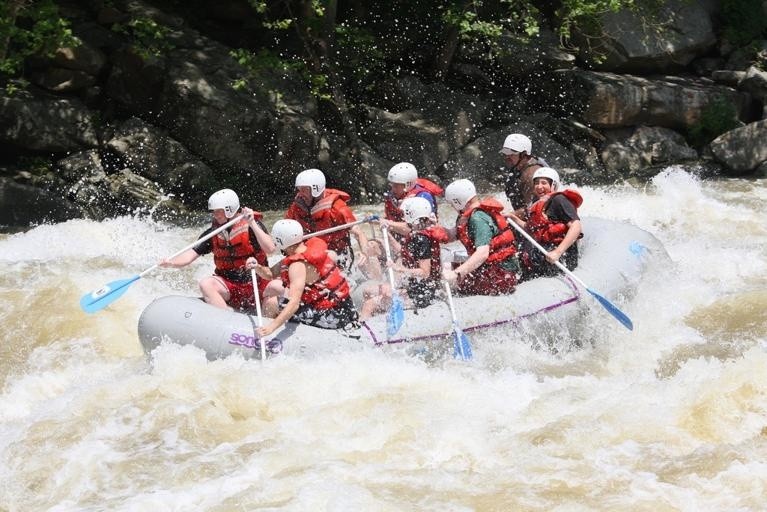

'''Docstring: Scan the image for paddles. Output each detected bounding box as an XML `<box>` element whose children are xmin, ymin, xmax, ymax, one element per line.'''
<box><xmin>79</xmin><ymin>216</ymin><xmax>244</xmax><ymax>312</ymax></box>
<box><xmin>382</xmin><ymin>227</ymin><xmax>404</xmax><ymax>336</ymax></box>
<box><xmin>507</xmin><ymin>218</ymin><xmax>633</xmax><ymax>331</ymax></box>
<box><xmin>437</xmin><ymin>242</ymin><xmax>473</xmax><ymax>363</ymax></box>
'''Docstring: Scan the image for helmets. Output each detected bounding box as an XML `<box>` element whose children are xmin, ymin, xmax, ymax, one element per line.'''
<box><xmin>531</xmin><ymin>166</ymin><xmax>561</xmax><ymax>194</ymax></box>
<box><xmin>294</xmin><ymin>168</ymin><xmax>328</xmax><ymax>199</ymax></box>
<box><xmin>501</xmin><ymin>132</ymin><xmax>532</xmax><ymax>158</ymax></box>
<box><xmin>444</xmin><ymin>176</ymin><xmax>477</xmax><ymax>212</ymax></box>
<box><xmin>206</xmin><ymin>187</ymin><xmax>240</xmax><ymax>219</ymax></box>
<box><xmin>268</xmin><ymin>218</ymin><xmax>304</xmax><ymax>250</ymax></box>
<box><xmin>385</xmin><ymin>161</ymin><xmax>418</xmax><ymax>193</ymax></box>
<box><xmin>398</xmin><ymin>195</ymin><xmax>432</xmax><ymax>224</ymax></box>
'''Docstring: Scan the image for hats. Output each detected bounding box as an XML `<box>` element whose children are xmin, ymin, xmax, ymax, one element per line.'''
<box><xmin>497</xmin><ymin>147</ymin><xmax>520</xmax><ymax>156</ymax></box>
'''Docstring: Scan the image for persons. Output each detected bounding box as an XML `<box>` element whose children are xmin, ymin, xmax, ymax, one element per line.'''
<box><xmin>361</xmin><ymin>195</ymin><xmax>448</xmax><ymax>321</ymax></box>
<box><xmin>432</xmin><ymin>179</ymin><xmax>518</xmax><ymax>296</ymax></box>
<box><xmin>498</xmin><ymin>133</ymin><xmax>552</xmax><ymax>210</ymax></box>
<box><xmin>502</xmin><ymin>167</ymin><xmax>582</xmax><ymax>283</ymax></box>
<box><xmin>158</xmin><ymin>189</ymin><xmax>276</xmax><ymax>312</ymax></box>
<box><xmin>243</xmin><ymin>216</ymin><xmax>357</xmax><ymax>338</ymax></box>
<box><xmin>365</xmin><ymin>162</ymin><xmax>443</xmax><ymax>263</ymax></box>
<box><xmin>283</xmin><ymin>169</ymin><xmax>367</xmax><ymax>275</ymax></box>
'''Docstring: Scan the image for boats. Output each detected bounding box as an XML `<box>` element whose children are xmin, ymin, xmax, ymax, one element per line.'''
<box><xmin>134</xmin><ymin>217</ymin><xmax>678</xmax><ymax>366</ymax></box>
